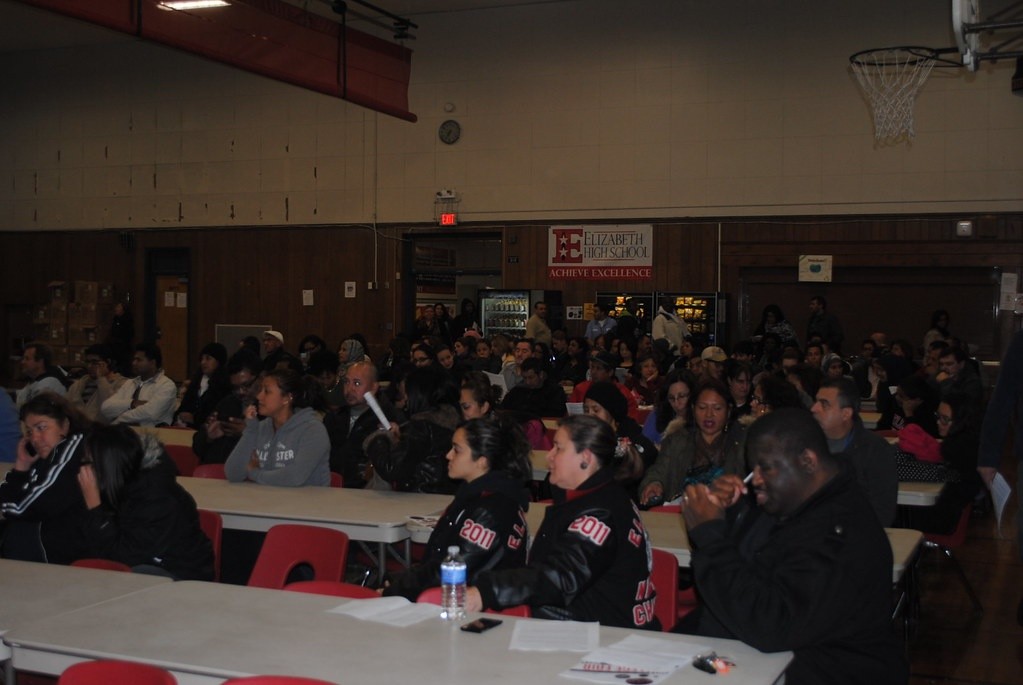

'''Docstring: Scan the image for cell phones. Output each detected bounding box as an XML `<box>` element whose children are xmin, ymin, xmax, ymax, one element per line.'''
<box><xmin>461</xmin><ymin>618</ymin><xmax>503</xmax><ymax>633</ymax></box>
<box><xmin>25</xmin><ymin>441</ymin><xmax>37</xmax><ymax>457</ymax></box>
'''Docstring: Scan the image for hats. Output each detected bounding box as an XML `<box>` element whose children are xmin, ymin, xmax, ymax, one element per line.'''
<box><xmin>201</xmin><ymin>342</ymin><xmax>227</xmax><ymax>364</ymax></box>
<box><xmin>591</xmin><ymin>351</ymin><xmax>617</xmax><ymax>370</ymax></box>
<box><xmin>584</xmin><ymin>382</ymin><xmax>628</xmax><ymax>422</ymax></box>
<box><xmin>701</xmin><ymin>346</ymin><xmax>728</xmax><ymax>362</ymax></box>
<box><xmin>263</xmin><ymin>331</ymin><xmax>285</xmax><ymax>345</ymax></box>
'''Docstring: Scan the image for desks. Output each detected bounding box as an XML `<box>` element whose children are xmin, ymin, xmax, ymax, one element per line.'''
<box><xmin>177</xmin><ymin>475</ymin><xmax>456</xmax><ymax>581</ymax></box>
<box><xmin>2</xmin><ymin>580</ymin><xmax>795</xmax><ymax>685</ymax></box>
<box><xmin>131</xmin><ymin>424</ymin><xmax>195</xmax><ymax>448</ymax></box>
<box><xmin>898</xmin><ymin>481</ymin><xmax>946</xmax><ymax>529</ymax></box>
<box><xmin>406</xmin><ymin>501</ymin><xmax>691</xmax><ymax>580</ymax></box>
<box><xmin>0</xmin><ymin>554</ymin><xmax>174</xmax><ymax>685</ymax></box>
<box><xmin>889</xmin><ymin>530</ymin><xmax>926</xmax><ymax>664</ymax></box>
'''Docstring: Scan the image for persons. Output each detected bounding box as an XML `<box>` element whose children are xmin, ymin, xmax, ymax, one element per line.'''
<box><xmin>583</xmin><ymin>303</ymin><xmax>617</xmax><ymax>339</ymax></box>
<box><xmin>0</xmin><ymin>330</ymin><xmax>992</xmax><ymax>684</ymax></box>
<box><xmin>752</xmin><ymin>305</ymin><xmax>797</xmax><ymax>343</ymax></box>
<box><xmin>434</xmin><ymin>303</ymin><xmax>454</xmax><ymax>336</ymax></box>
<box><xmin>416</xmin><ymin>306</ymin><xmax>452</xmax><ymax>347</ymax></box>
<box><xmin>923</xmin><ymin>311</ymin><xmax>949</xmax><ymax>364</ymax></box>
<box><xmin>112</xmin><ymin>303</ymin><xmax>135</xmax><ymax>353</ymax></box>
<box><xmin>526</xmin><ymin>301</ymin><xmax>553</xmax><ymax>348</ymax></box>
<box><xmin>453</xmin><ymin>298</ymin><xmax>483</xmax><ymax>338</ymax></box>
<box><xmin>974</xmin><ymin>328</ymin><xmax>1023</xmax><ymax>629</ymax></box>
<box><xmin>680</xmin><ymin>406</ymin><xmax>911</xmax><ymax>685</ymax></box>
<box><xmin>806</xmin><ymin>296</ymin><xmax>843</xmax><ymax>355</ymax></box>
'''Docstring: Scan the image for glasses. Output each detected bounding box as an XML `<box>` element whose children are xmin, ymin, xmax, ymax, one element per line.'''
<box><xmin>751</xmin><ymin>394</ymin><xmax>760</xmax><ymax>400</ymax></box>
<box><xmin>414</xmin><ymin>357</ymin><xmax>432</xmax><ymax>363</ymax></box>
<box><xmin>80</xmin><ymin>359</ymin><xmax>104</xmax><ymax>367</ymax></box>
<box><xmin>667</xmin><ymin>392</ymin><xmax>692</xmax><ymax>404</ymax></box>
<box><xmin>932</xmin><ymin>411</ymin><xmax>952</xmax><ymax>426</ymax></box>
<box><xmin>232</xmin><ymin>375</ymin><xmax>260</xmax><ymax>391</ymax></box>
<box><xmin>758</xmin><ymin>398</ymin><xmax>772</xmax><ymax>404</ymax></box>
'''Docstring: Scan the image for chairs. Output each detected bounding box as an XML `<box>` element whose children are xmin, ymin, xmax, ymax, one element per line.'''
<box><xmin>163</xmin><ymin>444</ymin><xmax>343</xmax><ymax>487</ymax></box>
<box><xmin>66</xmin><ymin>558</ymin><xmax>134</xmax><ymax>576</ymax></box>
<box><xmin>282</xmin><ymin>583</ymin><xmax>380</xmax><ymax>603</ymax></box>
<box><xmin>416</xmin><ymin>587</ymin><xmax>529</xmax><ymax>620</ymax></box>
<box><xmin>195</xmin><ymin>506</ymin><xmax>222</xmax><ymax>582</ymax></box>
<box><xmin>628</xmin><ymin>410</ymin><xmax>651</xmax><ymax>424</ymax></box>
<box><xmin>57</xmin><ymin>660</ymin><xmax>338</xmax><ymax>685</ymax></box>
<box><xmin>915</xmin><ymin>503</ymin><xmax>983</xmax><ymax>614</ymax></box>
<box><xmin>649</xmin><ymin>549</ymin><xmax>678</xmax><ymax>632</ymax></box>
<box><xmin>246</xmin><ymin>525</ymin><xmax>349</xmax><ymax>583</ymax></box>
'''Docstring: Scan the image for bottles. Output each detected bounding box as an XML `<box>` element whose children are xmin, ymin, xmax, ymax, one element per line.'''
<box><xmin>441</xmin><ymin>546</ymin><xmax>466</xmax><ymax>621</ymax></box>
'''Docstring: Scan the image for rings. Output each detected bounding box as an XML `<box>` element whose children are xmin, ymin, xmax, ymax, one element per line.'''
<box><xmin>684</xmin><ymin>497</ymin><xmax>688</xmax><ymax>499</ymax></box>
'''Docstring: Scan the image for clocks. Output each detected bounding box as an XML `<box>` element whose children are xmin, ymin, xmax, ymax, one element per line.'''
<box><xmin>439</xmin><ymin>120</ymin><xmax>460</xmax><ymax>143</ymax></box>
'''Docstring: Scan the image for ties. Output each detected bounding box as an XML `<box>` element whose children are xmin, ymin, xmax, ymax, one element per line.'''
<box><xmin>132</xmin><ymin>383</ymin><xmax>143</xmax><ymax>401</ymax></box>
